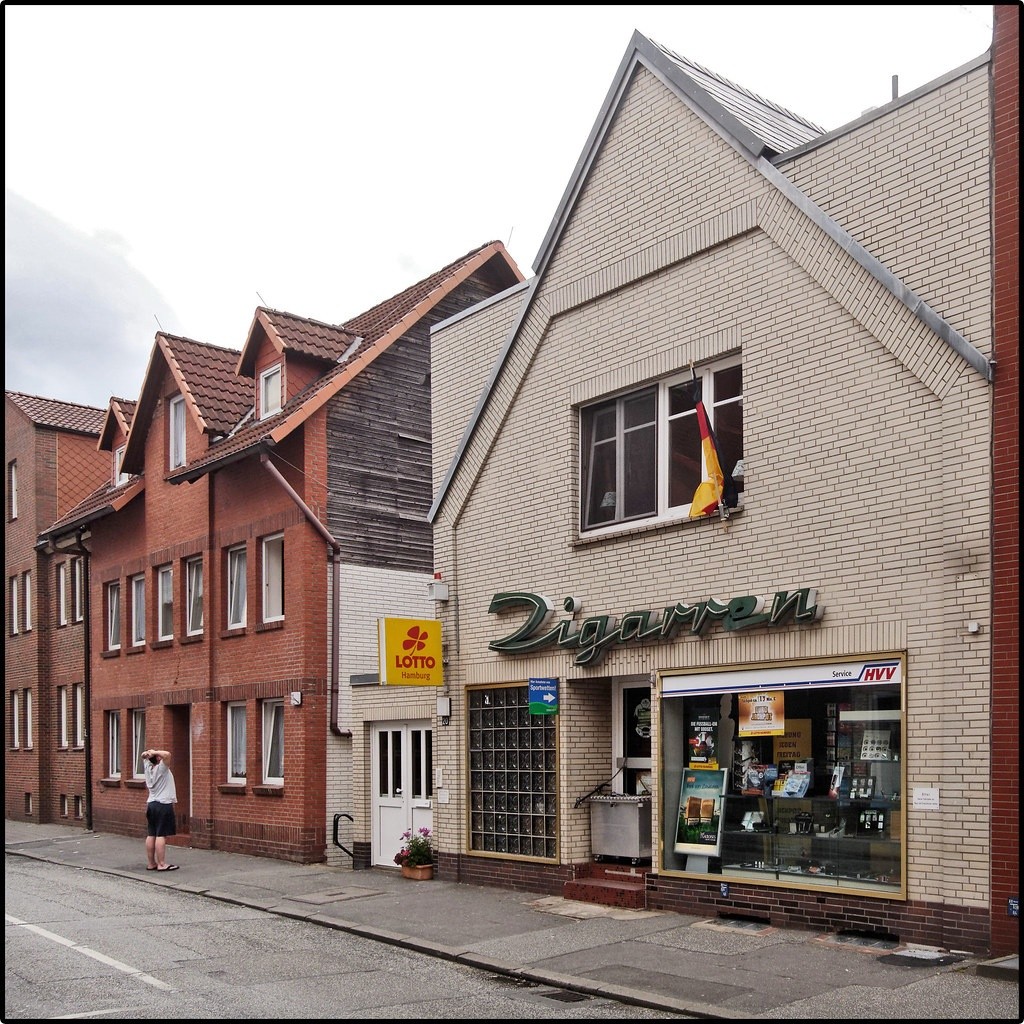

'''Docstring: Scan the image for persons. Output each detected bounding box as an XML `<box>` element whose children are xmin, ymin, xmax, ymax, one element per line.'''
<box><xmin>140</xmin><ymin>748</ymin><xmax>180</xmax><ymax>872</ymax></box>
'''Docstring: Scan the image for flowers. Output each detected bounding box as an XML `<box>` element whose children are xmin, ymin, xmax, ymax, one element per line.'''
<box><xmin>393</xmin><ymin>826</ymin><xmax>432</xmax><ymax>867</ymax></box>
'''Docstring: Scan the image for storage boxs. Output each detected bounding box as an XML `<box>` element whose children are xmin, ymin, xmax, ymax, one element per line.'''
<box><xmin>773</xmin><ymin>757</ymin><xmax>814</xmax><ymax>798</ymax></box>
<box><xmin>762</xmin><ymin>834</ymin><xmax>819</xmax><ymax>866</ymax></box>
<box><xmin>758</xmin><ymin>797</ymin><xmax>838</xmax><ymax>835</ymax></box>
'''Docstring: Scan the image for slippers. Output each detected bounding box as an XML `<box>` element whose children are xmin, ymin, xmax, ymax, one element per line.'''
<box><xmin>147</xmin><ymin>865</ymin><xmax>157</xmax><ymax>870</ymax></box>
<box><xmin>158</xmin><ymin>864</ymin><xmax>179</xmax><ymax>871</ymax></box>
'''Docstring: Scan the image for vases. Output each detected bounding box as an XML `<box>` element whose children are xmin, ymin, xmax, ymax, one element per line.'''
<box><xmin>402</xmin><ymin>865</ymin><xmax>433</xmax><ymax>880</ymax></box>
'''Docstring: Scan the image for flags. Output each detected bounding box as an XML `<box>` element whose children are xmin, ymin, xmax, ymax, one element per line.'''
<box><xmin>687</xmin><ymin>364</ymin><xmax>724</xmax><ymax>518</ymax></box>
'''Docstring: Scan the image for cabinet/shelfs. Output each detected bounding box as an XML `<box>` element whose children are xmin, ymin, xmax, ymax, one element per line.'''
<box><xmin>718</xmin><ymin>794</ymin><xmax>901</xmax><ymax>865</ymax></box>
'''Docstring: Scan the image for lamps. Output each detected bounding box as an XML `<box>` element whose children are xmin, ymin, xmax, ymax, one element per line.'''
<box><xmin>436</xmin><ymin>697</ymin><xmax>452</xmax><ymax>726</ymax></box>
